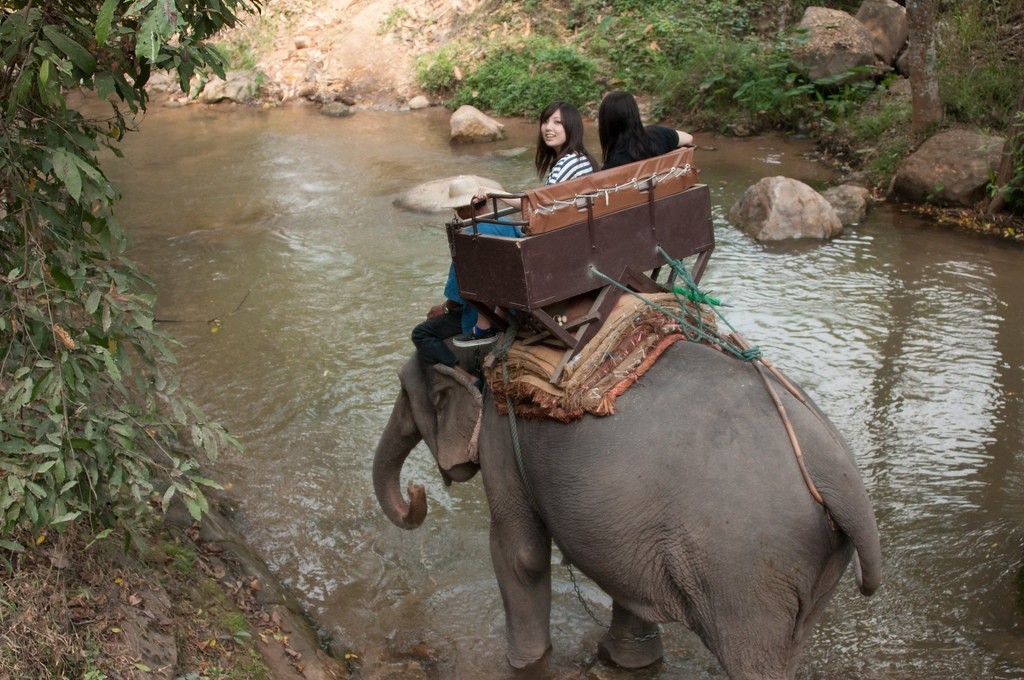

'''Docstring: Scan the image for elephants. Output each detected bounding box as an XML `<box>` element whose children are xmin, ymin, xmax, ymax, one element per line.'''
<box><xmin>371</xmin><ymin>275</ymin><xmax>883</xmax><ymax>679</ymax></box>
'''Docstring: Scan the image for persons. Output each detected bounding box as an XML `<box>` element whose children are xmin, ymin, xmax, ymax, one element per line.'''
<box><xmin>452</xmin><ymin>101</ymin><xmax>603</xmax><ymax>348</ymax></box>
<box><xmin>410</xmin><ymin>173</ymin><xmax>524</xmax><ymax>390</ymax></box>
<box><xmin>597</xmin><ymin>90</ymin><xmax>695</xmax><ymax>170</ymax></box>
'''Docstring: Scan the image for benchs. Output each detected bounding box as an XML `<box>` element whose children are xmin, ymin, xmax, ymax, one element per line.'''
<box><xmin>444</xmin><ymin>143</ymin><xmax>717</xmax><ymax>382</ymax></box>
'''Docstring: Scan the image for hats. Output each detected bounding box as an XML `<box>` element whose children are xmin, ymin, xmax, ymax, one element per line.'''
<box><xmin>441</xmin><ymin>178</ymin><xmax>488</xmax><ymax>207</ymax></box>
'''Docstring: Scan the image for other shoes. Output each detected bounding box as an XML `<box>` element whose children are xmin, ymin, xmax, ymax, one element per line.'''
<box><xmin>471</xmin><ymin>378</ymin><xmax>481</xmax><ymax>390</ymax></box>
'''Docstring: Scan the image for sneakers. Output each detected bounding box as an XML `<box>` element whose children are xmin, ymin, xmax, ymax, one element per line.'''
<box><xmin>453</xmin><ymin>324</ymin><xmax>498</xmax><ymax>347</ymax></box>
